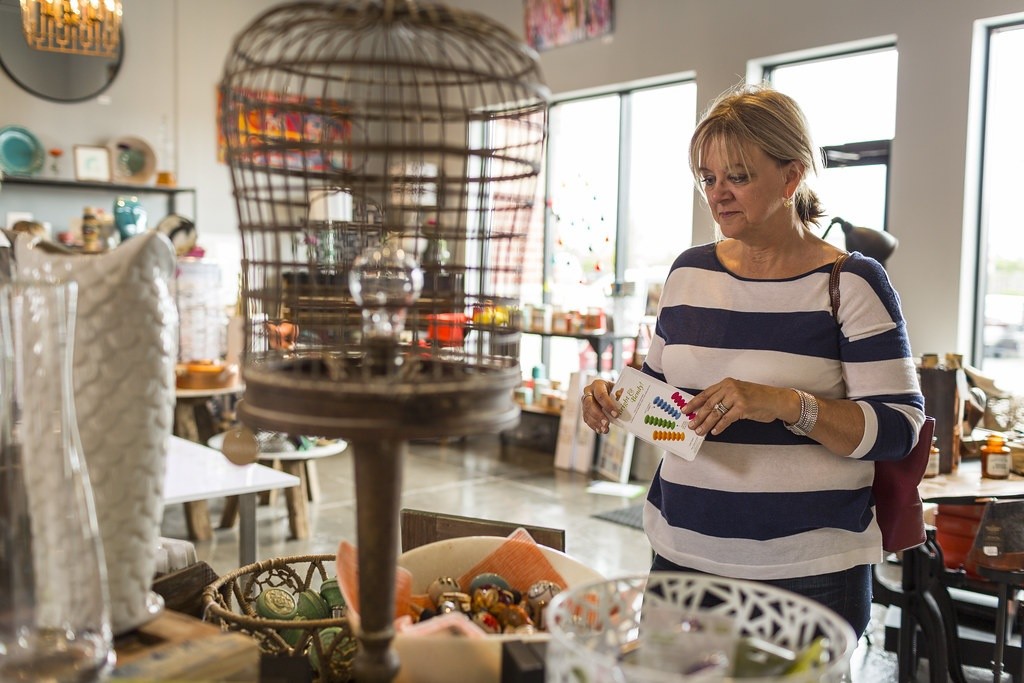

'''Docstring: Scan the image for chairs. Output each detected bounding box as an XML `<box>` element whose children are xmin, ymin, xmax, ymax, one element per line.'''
<box><xmin>399</xmin><ymin>508</ymin><xmax>566</xmax><ymax>557</ymax></box>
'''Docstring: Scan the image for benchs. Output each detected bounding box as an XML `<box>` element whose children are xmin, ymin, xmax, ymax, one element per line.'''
<box><xmin>884</xmin><ymin>504</ymin><xmax>1024</xmax><ymax>674</ymax></box>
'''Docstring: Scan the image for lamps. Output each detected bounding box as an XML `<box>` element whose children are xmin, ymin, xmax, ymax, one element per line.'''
<box><xmin>820</xmin><ymin>216</ymin><xmax>899</xmax><ymax>266</ymax></box>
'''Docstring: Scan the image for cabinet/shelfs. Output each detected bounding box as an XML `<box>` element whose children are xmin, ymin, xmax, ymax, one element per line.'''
<box><xmin>476</xmin><ymin>324</ymin><xmax>644</xmax><ymax>480</ymax></box>
<box><xmin>0</xmin><ymin>175</ymin><xmax>198</xmax><ymax>360</ymax></box>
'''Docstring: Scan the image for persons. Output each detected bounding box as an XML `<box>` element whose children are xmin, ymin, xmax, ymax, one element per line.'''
<box><xmin>580</xmin><ymin>84</ymin><xmax>927</xmax><ymax>644</ymax></box>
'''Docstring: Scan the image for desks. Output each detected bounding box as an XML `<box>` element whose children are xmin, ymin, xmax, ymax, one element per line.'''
<box><xmin>898</xmin><ymin>451</ymin><xmax>1024</xmax><ymax>683</ymax></box>
<box><xmin>164</xmin><ymin>434</ymin><xmax>301</xmax><ymax>608</ymax></box>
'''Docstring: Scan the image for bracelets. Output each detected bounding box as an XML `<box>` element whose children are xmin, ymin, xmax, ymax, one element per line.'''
<box><xmin>784</xmin><ymin>386</ymin><xmax>818</xmax><ymax>436</ymax></box>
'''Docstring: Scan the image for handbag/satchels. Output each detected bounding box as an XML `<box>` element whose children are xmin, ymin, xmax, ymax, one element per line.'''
<box><xmin>829</xmin><ymin>253</ymin><xmax>935</xmax><ymax>552</ymax></box>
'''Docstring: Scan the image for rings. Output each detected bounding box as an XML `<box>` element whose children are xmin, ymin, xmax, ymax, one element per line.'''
<box><xmin>581</xmin><ymin>392</ymin><xmax>591</xmax><ymax>401</ymax></box>
<box><xmin>715</xmin><ymin>402</ymin><xmax>728</xmax><ymax>414</ymax></box>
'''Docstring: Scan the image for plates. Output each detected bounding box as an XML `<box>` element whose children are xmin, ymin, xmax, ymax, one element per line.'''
<box><xmin>0</xmin><ymin>125</ymin><xmax>45</xmax><ymax>178</ymax></box>
<box><xmin>106</xmin><ymin>133</ymin><xmax>156</xmax><ymax>184</ymax></box>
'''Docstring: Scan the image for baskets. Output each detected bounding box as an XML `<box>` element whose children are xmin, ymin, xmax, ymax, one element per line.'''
<box><xmin>545</xmin><ymin>571</ymin><xmax>858</xmax><ymax>683</ymax></box>
<box><xmin>200</xmin><ymin>554</ymin><xmax>358</xmax><ymax>683</ymax></box>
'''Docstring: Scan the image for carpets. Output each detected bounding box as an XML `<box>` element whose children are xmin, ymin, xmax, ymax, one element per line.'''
<box><xmin>594</xmin><ymin>502</ymin><xmax>648</xmax><ymax>532</ymax></box>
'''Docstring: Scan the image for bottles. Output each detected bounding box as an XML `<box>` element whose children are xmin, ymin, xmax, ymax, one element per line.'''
<box><xmin>923</xmin><ymin>435</ymin><xmax>939</xmax><ymax>478</ymax></box>
<box><xmin>0</xmin><ymin>221</ymin><xmax>119</xmax><ymax>683</ymax></box>
<box><xmin>82</xmin><ymin>207</ymin><xmax>99</xmax><ymax>251</ymax></box>
<box><xmin>980</xmin><ymin>436</ymin><xmax>1012</xmax><ymax>480</ymax></box>
